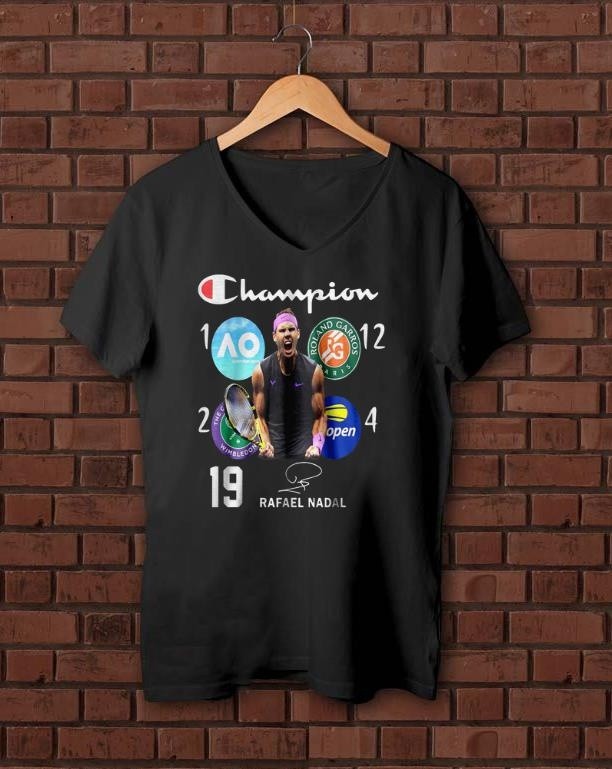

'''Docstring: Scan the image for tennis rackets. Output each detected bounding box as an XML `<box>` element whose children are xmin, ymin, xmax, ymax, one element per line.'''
<box><xmin>224</xmin><ymin>383</ymin><xmax>270</xmax><ymax>455</ymax></box>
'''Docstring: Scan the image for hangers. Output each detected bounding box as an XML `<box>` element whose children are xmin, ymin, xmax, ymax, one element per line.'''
<box><xmin>217</xmin><ymin>24</ymin><xmax>390</xmax><ymax>158</ymax></box>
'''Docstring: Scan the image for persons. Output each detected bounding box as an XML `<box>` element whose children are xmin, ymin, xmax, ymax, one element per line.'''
<box><xmin>252</xmin><ymin>309</ymin><xmax>327</xmax><ymax>461</ymax></box>
